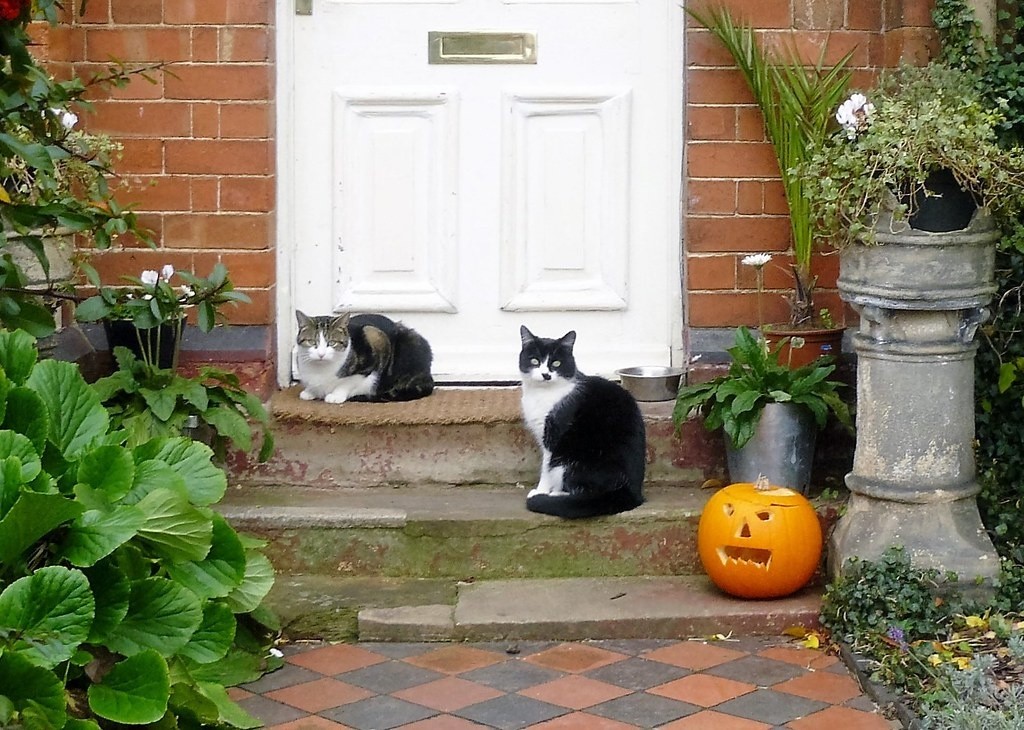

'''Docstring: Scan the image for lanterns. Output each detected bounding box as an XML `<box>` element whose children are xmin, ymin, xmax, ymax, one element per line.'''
<box><xmin>698</xmin><ymin>471</ymin><xmax>823</xmax><ymax>601</ymax></box>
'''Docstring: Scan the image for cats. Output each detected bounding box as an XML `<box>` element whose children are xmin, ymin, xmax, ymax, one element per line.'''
<box><xmin>519</xmin><ymin>324</ymin><xmax>652</xmax><ymax>522</ymax></box>
<box><xmin>293</xmin><ymin>308</ymin><xmax>435</xmax><ymax>405</ymax></box>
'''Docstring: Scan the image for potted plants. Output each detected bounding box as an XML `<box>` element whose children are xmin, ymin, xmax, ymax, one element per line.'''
<box><xmin>680</xmin><ymin>0</ymin><xmax>849</xmax><ymax>372</ymax></box>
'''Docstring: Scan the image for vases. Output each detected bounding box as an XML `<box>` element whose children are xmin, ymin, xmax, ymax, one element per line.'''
<box><xmin>104</xmin><ymin>316</ymin><xmax>189</xmax><ymax>370</ymax></box>
<box><xmin>724</xmin><ymin>402</ymin><xmax>814</xmax><ymax>497</ymax></box>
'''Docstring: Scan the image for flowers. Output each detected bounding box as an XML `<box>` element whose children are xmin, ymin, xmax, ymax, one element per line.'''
<box><xmin>665</xmin><ymin>256</ymin><xmax>858</xmax><ymax>447</ymax></box>
<box><xmin>73</xmin><ymin>262</ymin><xmax>251</xmax><ymax>334</ymax></box>
